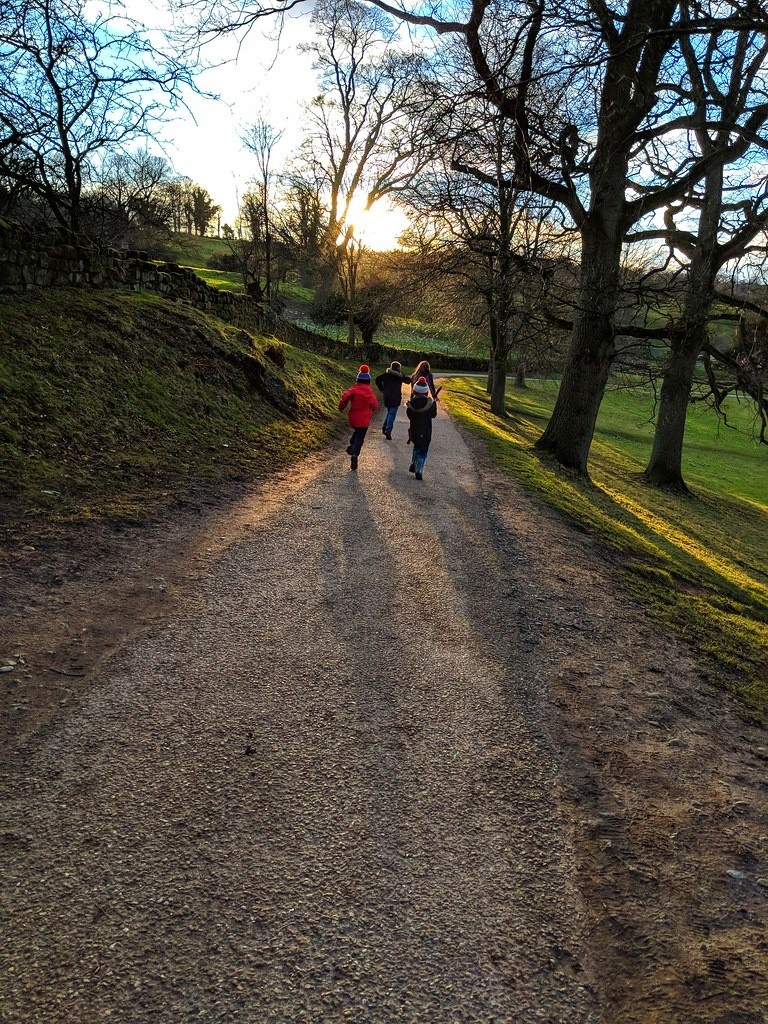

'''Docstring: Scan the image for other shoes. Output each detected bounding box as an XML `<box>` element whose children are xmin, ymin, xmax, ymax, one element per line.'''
<box><xmin>346</xmin><ymin>446</ymin><xmax>353</xmax><ymax>455</ymax></box>
<box><xmin>351</xmin><ymin>454</ymin><xmax>358</xmax><ymax>470</ymax></box>
<box><xmin>409</xmin><ymin>464</ymin><xmax>416</xmax><ymax>473</ymax></box>
<box><xmin>415</xmin><ymin>472</ymin><xmax>422</xmax><ymax>480</ymax></box>
<box><xmin>382</xmin><ymin>426</ymin><xmax>392</xmax><ymax>440</ymax></box>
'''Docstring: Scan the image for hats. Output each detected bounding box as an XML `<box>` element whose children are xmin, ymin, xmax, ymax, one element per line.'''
<box><xmin>413</xmin><ymin>377</ymin><xmax>429</xmax><ymax>396</ymax></box>
<box><xmin>356</xmin><ymin>365</ymin><xmax>370</xmax><ymax>384</ymax></box>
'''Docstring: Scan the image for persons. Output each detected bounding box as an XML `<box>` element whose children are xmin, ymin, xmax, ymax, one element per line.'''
<box><xmin>405</xmin><ymin>361</ymin><xmax>441</xmax><ymax>480</ymax></box>
<box><xmin>339</xmin><ymin>365</ymin><xmax>379</xmax><ymax>470</ymax></box>
<box><xmin>375</xmin><ymin>360</ymin><xmax>411</xmax><ymax>440</ymax></box>
